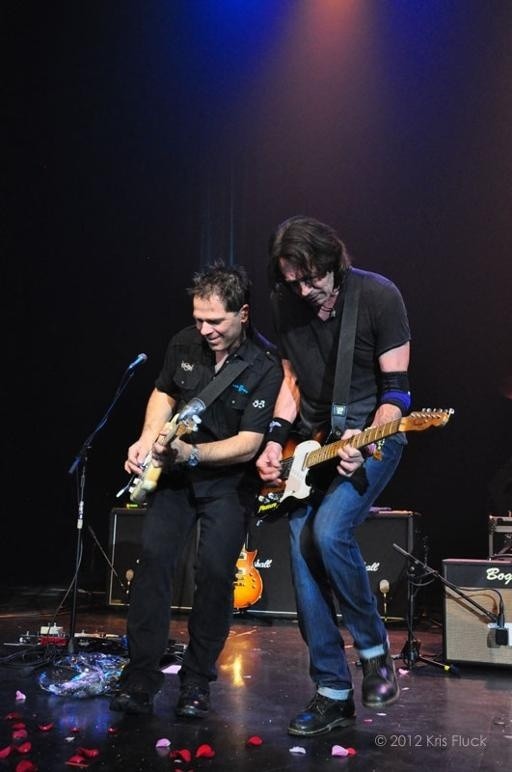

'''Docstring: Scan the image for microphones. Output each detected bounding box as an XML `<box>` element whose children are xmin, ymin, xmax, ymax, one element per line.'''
<box><xmin>490</xmin><ymin>602</ymin><xmax>512</xmax><ymax>647</ymax></box>
<box><xmin>124</xmin><ymin>350</ymin><xmax>152</xmax><ymax>375</ymax></box>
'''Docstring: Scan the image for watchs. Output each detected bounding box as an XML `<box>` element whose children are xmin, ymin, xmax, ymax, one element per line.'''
<box><xmin>187</xmin><ymin>443</ymin><xmax>200</xmax><ymax>467</ymax></box>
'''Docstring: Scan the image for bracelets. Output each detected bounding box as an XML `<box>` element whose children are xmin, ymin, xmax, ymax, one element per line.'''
<box><xmin>365</xmin><ymin>425</ymin><xmax>385</xmax><ymax>451</ymax></box>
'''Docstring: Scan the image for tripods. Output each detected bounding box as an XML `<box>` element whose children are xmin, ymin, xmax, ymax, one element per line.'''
<box><xmin>58</xmin><ymin>350</ymin><xmax>134</xmax><ymax>660</ymax></box>
<box><xmin>353</xmin><ymin>531</ymin><xmax>509</xmax><ymax>684</ymax></box>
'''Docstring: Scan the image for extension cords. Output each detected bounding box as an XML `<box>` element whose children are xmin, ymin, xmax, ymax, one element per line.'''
<box><xmin>73</xmin><ymin>629</ymin><xmax>118</xmax><ymax>640</ymax></box>
<box><xmin>39</xmin><ymin>619</ymin><xmax>62</xmax><ymax>636</ymax></box>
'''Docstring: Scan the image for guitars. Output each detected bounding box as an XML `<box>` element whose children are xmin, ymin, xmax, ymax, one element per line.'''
<box><xmin>233</xmin><ymin>530</ymin><xmax>262</xmax><ymax>610</ymax></box>
<box><xmin>255</xmin><ymin>406</ymin><xmax>459</xmax><ymax>513</ymax></box>
<box><xmin>129</xmin><ymin>409</ymin><xmax>202</xmax><ymax>518</ymax></box>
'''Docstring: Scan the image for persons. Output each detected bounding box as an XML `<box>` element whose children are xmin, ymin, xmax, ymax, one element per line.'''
<box><xmin>111</xmin><ymin>262</ymin><xmax>284</xmax><ymax>715</ymax></box>
<box><xmin>255</xmin><ymin>214</ymin><xmax>410</xmax><ymax>737</ymax></box>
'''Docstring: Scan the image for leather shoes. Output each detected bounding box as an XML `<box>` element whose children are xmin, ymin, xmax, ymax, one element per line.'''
<box><xmin>109</xmin><ymin>689</ymin><xmax>155</xmax><ymax>718</ymax></box>
<box><xmin>175</xmin><ymin>680</ymin><xmax>211</xmax><ymax>722</ymax></box>
<box><xmin>287</xmin><ymin>691</ymin><xmax>357</xmax><ymax>738</ymax></box>
<box><xmin>360</xmin><ymin>650</ymin><xmax>400</xmax><ymax>710</ymax></box>
